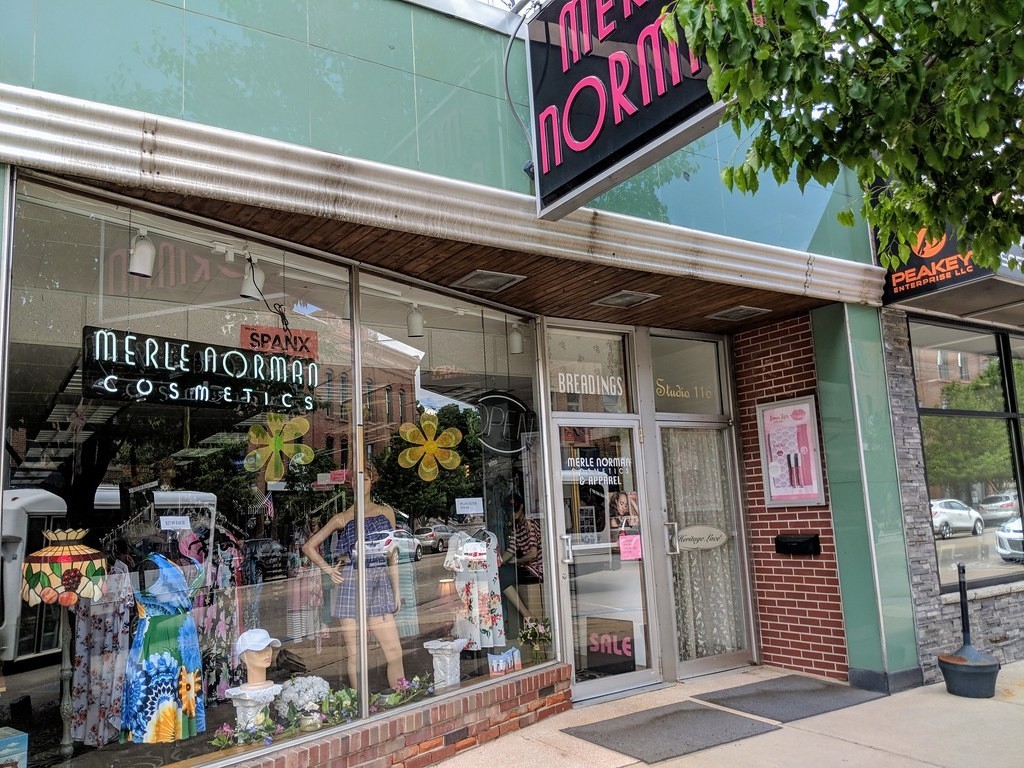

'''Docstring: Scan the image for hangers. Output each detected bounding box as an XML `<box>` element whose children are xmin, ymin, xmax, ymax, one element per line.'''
<box><xmin>99</xmin><ymin>507</ymin><xmax>232</xmax><ymax>575</ymax></box>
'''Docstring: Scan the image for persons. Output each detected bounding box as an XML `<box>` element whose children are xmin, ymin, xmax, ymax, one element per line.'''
<box><xmin>443</xmin><ymin>512</ymin><xmax>506</xmax><ymax>651</ymax></box>
<box><xmin>610</xmin><ymin>492</ymin><xmax>633</xmax><ymax>527</ymax></box>
<box><xmin>302</xmin><ymin>466</ymin><xmax>406</xmax><ymax>690</ymax></box>
<box><xmin>498</xmin><ymin>494</ymin><xmax>544</xmax><ymax>625</ymax></box>
<box><xmin>119</xmin><ymin>541</ymin><xmax>205</xmax><ymax>745</ymax></box>
<box><xmin>234</xmin><ymin>627</ymin><xmax>281</xmax><ymax>690</ymax></box>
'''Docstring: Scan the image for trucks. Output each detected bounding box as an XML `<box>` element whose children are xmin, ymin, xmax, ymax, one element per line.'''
<box><xmin>0</xmin><ymin>485</ymin><xmax>216</xmax><ymax>663</ymax></box>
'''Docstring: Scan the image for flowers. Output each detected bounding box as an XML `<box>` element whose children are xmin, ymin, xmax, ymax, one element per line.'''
<box><xmin>210</xmin><ymin>671</ymin><xmax>436</xmax><ymax>750</ymax></box>
<box><xmin>517</xmin><ymin>618</ymin><xmax>552</xmax><ymax>664</ymax></box>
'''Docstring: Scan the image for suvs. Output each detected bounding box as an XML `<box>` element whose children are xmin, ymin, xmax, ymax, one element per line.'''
<box><xmin>350</xmin><ymin>529</ymin><xmax>423</xmax><ymax>569</ymax></box>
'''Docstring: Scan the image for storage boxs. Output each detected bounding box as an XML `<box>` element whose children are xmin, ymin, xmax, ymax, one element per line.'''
<box><xmin>0</xmin><ymin>727</ymin><xmax>28</xmax><ymax>768</ymax></box>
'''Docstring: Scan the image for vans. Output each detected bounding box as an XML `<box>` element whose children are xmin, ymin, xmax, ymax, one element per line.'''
<box><xmin>561</xmin><ymin>470</ymin><xmax>614</xmax><ymax>580</ymax></box>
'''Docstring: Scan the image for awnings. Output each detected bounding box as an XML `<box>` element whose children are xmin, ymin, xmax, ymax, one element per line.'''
<box><xmin>873</xmin><ymin>215</ymin><xmax>1024</xmax><ymax>307</ymax></box>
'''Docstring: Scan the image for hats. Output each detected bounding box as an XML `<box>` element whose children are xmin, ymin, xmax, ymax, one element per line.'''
<box><xmin>235</xmin><ymin>629</ymin><xmax>282</xmax><ymax>656</ymax></box>
<box><xmin>504</xmin><ymin>493</ymin><xmax>522</xmax><ymax>513</ymax></box>
<box><xmin>346</xmin><ymin>460</ymin><xmax>379</xmax><ymax>483</ymax></box>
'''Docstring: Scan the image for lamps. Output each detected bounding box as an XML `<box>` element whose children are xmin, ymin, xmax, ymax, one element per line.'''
<box><xmin>406</xmin><ymin>303</ymin><xmax>427</xmax><ymax>337</ymax></box>
<box><xmin>239</xmin><ymin>255</ymin><xmax>265</xmax><ymax>303</ymax></box>
<box><xmin>127</xmin><ymin>229</ymin><xmax>157</xmax><ymax>278</ymax></box>
<box><xmin>508</xmin><ymin>323</ymin><xmax>524</xmax><ymax>354</ymax></box>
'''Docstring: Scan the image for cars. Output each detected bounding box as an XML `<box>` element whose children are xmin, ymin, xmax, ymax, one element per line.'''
<box><xmin>415</xmin><ymin>524</ymin><xmax>457</xmax><ymax>553</ymax></box>
<box><xmin>233</xmin><ymin>538</ymin><xmax>300</xmax><ymax>585</ymax></box>
<box><xmin>930</xmin><ymin>499</ymin><xmax>985</xmax><ymax>540</ymax></box>
<box><xmin>979</xmin><ymin>494</ymin><xmax>1018</xmax><ymax>526</ymax></box>
<box><xmin>994</xmin><ymin>518</ymin><xmax>1024</xmax><ymax>563</ymax></box>
<box><xmin>609</xmin><ymin>515</ymin><xmax>640</xmax><ymax>551</ymax></box>
